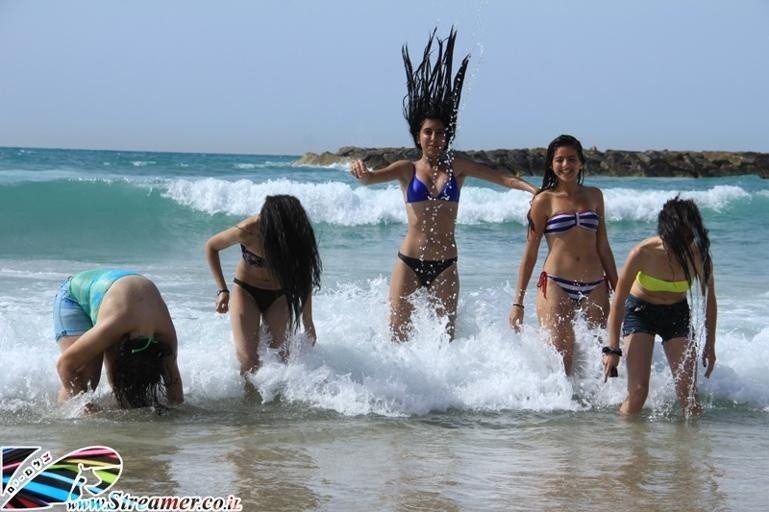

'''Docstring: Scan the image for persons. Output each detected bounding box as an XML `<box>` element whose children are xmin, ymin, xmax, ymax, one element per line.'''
<box><xmin>52</xmin><ymin>268</ymin><xmax>183</xmax><ymax>419</ymax></box>
<box><xmin>207</xmin><ymin>195</ymin><xmax>322</xmax><ymax>403</ymax></box>
<box><xmin>509</xmin><ymin>133</ymin><xmax>618</xmax><ymax>378</ymax></box>
<box><xmin>601</xmin><ymin>194</ymin><xmax>718</xmax><ymax>420</ymax></box>
<box><xmin>353</xmin><ymin>22</ymin><xmax>539</xmax><ymax>347</ymax></box>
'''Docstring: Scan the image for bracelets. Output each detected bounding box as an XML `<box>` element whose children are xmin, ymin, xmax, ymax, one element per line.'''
<box><xmin>602</xmin><ymin>345</ymin><xmax>622</xmax><ymax>356</ymax></box>
<box><xmin>512</xmin><ymin>303</ymin><xmax>525</xmax><ymax>309</ymax></box>
<box><xmin>216</xmin><ymin>289</ymin><xmax>229</xmax><ymax>296</ymax></box>
